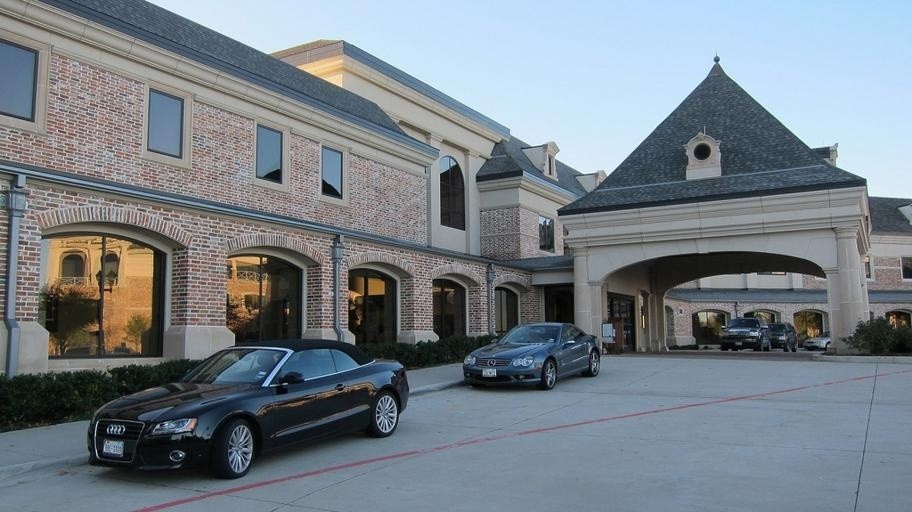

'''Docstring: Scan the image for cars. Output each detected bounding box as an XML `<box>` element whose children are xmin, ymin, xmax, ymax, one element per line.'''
<box><xmin>463</xmin><ymin>322</ymin><xmax>601</xmax><ymax>389</ymax></box>
<box><xmin>87</xmin><ymin>338</ymin><xmax>409</xmax><ymax>479</ymax></box>
<box><xmin>721</xmin><ymin>317</ymin><xmax>831</xmax><ymax>352</ymax></box>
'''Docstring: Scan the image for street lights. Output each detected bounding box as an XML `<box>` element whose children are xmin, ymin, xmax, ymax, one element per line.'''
<box><xmin>96</xmin><ymin>252</ymin><xmax>119</xmax><ymax>318</ymax></box>
